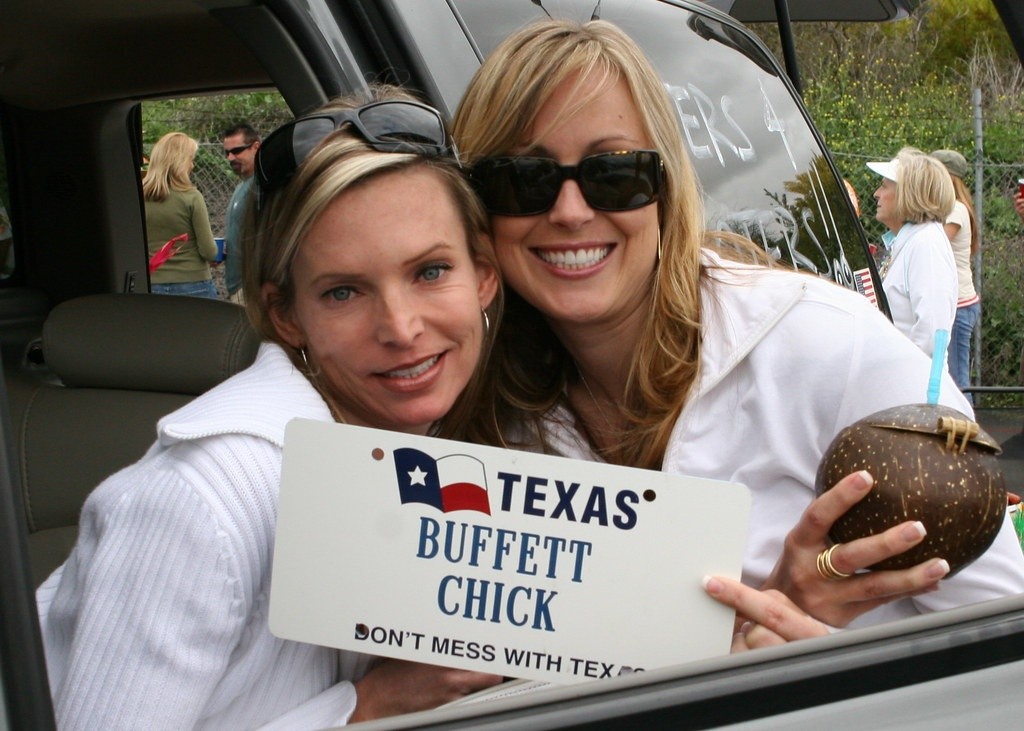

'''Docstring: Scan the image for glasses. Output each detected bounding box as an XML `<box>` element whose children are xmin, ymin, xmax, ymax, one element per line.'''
<box><xmin>464</xmin><ymin>150</ymin><xmax>665</xmax><ymax>217</ymax></box>
<box><xmin>224</xmin><ymin>144</ymin><xmax>252</xmax><ymax>156</ymax></box>
<box><xmin>253</xmin><ymin>99</ymin><xmax>455</xmax><ymax>226</ymax></box>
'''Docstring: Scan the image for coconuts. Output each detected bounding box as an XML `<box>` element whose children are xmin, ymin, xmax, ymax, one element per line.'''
<box><xmin>815</xmin><ymin>403</ymin><xmax>1007</xmax><ymax>581</ymax></box>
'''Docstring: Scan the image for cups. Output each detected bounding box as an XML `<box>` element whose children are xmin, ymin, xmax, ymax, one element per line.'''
<box><xmin>1017</xmin><ymin>178</ymin><xmax>1024</xmax><ymax>200</ymax></box>
<box><xmin>213</xmin><ymin>238</ymin><xmax>225</xmax><ymax>262</ymax></box>
<box><xmin>869</xmin><ymin>243</ymin><xmax>877</xmax><ymax>255</ymax></box>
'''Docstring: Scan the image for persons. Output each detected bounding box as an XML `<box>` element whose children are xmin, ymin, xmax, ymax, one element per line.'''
<box><xmin>34</xmin><ymin>84</ymin><xmax>504</xmax><ymax>729</ymax></box>
<box><xmin>931</xmin><ymin>150</ymin><xmax>981</xmax><ymax>409</ymax></box>
<box><xmin>454</xmin><ymin>19</ymin><xmax>1024</xmax><ymax>657</ymax></box>
<box><xmin>224</xmin><ymin>122</ymin><xmax>262</xmax><ymax>304</ymax></box>
<box><xmin>1013</xmin><ymin>192</ymin><xmax>1024</xmax><ymax>221</ymax></box>
<box><xmin>141</xmin><ymin>132</ymin><xmax>218</xmax><ymax>299</ymax></box>
<box><xmin>866</xmin><ymin>147</ymin><xmax>958</xmax><ymax>380</ymax></box>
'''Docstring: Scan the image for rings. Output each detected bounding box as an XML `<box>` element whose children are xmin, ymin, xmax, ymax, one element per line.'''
<box><xmin>817</xmin><ymin>543</ymin><xmax>853</xmax><ymax>580</ymax></box>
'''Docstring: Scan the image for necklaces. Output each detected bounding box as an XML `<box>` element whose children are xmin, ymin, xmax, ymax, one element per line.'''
<box><xmin>577</xmin><ymin>371</ymin><xmax>616</xmax><ymax>437</ymax></box>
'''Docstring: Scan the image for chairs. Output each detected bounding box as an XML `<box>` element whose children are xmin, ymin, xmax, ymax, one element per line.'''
<box><xmin>0</xmin><ymin>291</ymin><xmax>261</xmax><ymax>581</ymax></box>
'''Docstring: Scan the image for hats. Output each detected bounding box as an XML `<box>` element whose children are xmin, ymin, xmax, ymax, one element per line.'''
<box><xmin>930</xmin><ymin>150</ymin><xmax>967</xmax><ymax>178</ymax></box>
<box><xmin>866</xmin><ymin>158</ymin><xmax>903</xmax><ymax>183</ymax></box>
